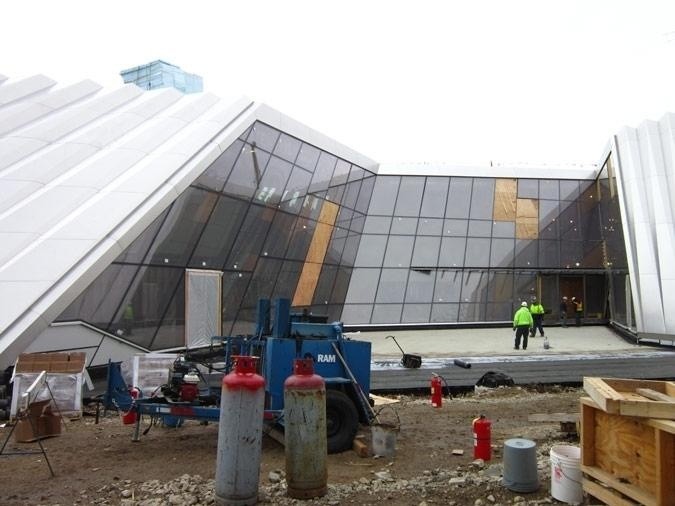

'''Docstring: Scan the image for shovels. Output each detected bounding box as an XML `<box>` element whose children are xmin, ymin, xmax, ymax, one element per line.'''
<box><xmin>331</xmin><ymin>342</ymin><xmax>397</xmax><ymax>457</ymax></box>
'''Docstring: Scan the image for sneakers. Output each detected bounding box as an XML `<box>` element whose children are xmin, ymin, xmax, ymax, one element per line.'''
<box><xmin>530</xmin><ymin>333</ymin><xmax>544</xmax><ymax>337</ymax></box>
<box><xmin>514</xmin><ymin>346</ymin><xmax>526</xmax><ymax>349</ymax></box>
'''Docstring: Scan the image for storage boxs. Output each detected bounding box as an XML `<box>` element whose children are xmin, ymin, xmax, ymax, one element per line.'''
<box><xmin>15</xmin><ymin>398</ymin><xmax>63</xmax><ymax>443</ymax></box>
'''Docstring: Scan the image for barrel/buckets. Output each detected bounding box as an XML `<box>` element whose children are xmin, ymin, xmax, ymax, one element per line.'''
<box><xmin>503</xmin><ymin>438</ymin><xmax>539</xmax><ymax>493</ymax></box>
<box><xmin>368</xmin><ymin>405</ymin><xmax>401</xmax><ymax>456</ymax></box>
<box><xmin>549</xmin><ymin>444</ymin><xmax>584</xmax><ymax>505</ymax></box>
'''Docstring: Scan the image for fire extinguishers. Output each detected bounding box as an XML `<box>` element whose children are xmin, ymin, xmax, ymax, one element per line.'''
<box><xmin>122</xmin><ymin>383</ymin><xmax>139</xmax><ymax>424</ymax></box>
<box><xmin>431</xmin><ymin>372</ymin><xmax>442</xmax><ymax>408</ymax></box>
<box><xmin>472</xmin><ymin>414</ymin><xmax>491</xmax><ymax>461</ymax></box>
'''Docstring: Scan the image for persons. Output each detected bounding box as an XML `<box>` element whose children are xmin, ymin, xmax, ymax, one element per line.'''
<box><xmin>559</xmin><ymin>297</ymin><xmax>570</xmax><ymax>329</ymax></box>
<box><xmin>529</xmin><ymin>296</ymin><xmax>546</xmax><ymax>338</ymax></box>
<box><xmin>571</xmin><ymin>297</ymin><xmax>584</xmax><ymax>328</ymax></box>
<box><xmin>512</xmin><ymin>301</ymin><xmax>533</xmax><ymax>350</ymax></box>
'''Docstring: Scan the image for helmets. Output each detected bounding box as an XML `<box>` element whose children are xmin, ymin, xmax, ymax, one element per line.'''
<box><xmin>563</xmin><ymin>297</ymin><xmax>567</xmax><ymax>300</ymax></box>
<box><xmin>572</xmin><ymin>297</ymin><xmax>576</xmax><ymax>301</ymax></box>
<box><xmin>521</xmin><ymin>302</ymin><xmax>528</xmax><ymax>307</ymax></box>
